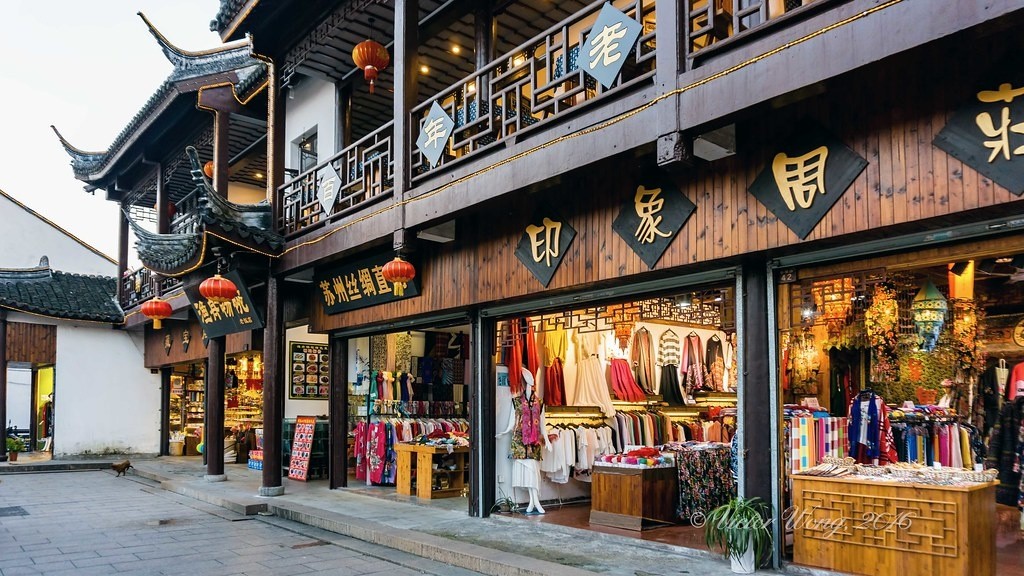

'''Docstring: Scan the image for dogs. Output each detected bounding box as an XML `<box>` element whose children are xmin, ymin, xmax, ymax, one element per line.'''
<box><xmin>110</xmin><ymin>459</ymin><xmax>134</xmax><ymax>477</ymax></box>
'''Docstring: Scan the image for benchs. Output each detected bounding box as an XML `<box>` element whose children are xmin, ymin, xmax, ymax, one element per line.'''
<box><xmin>534</xmin><ymin>95</ymin><xmax>572</xmax><ymax>121</ymax></box>
<box><xmin>486</xmin><ymin>115</ymin><xmax>527</xmax><ymax>141</ymax></box>
<box><xmin>350</xmin><ymin>190</ymin><xmax>365</xmax><ymax>204</ymax></box>
<box><xmin>701</xmin><ymin>7</ymin><xmax>745</xmax><ymax>49</ymax></box>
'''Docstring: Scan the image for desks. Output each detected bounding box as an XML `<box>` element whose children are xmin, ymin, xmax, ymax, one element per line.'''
<box><xmin>284</xmin><ymin>40</ymin><xmax>657</xmax><ymax>219</ymax></box>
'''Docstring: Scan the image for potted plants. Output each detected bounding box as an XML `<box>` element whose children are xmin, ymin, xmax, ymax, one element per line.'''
<box><xmin>489</xmin><ymin>486</ymin><xmax>523</xmax><ymax>516</ymax></box>
<box><xmin>5</xmin><ymin>434</ymin><xmax>28</xmax><ymax>461</ymax></box>
<box><xmin>700</xmin><ymin>493</ymin><xmax>776</xmax><ymax>573</ymax></box>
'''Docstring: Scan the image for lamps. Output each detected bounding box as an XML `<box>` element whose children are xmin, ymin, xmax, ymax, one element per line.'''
<box><xmin>677</xmin><ymin>290</ymin><xmax>721</xmax><ymax>311</ymax></box>
<box><xmin>948</xmin><ymin>259</ymin><xmax>975</xmax><ymax>347</ymax></box>
<box><xmin>606</xmin><ymin>301</ymin><xmax>638</xmax><ymax>355</ymax></box>
<box><xmin>810</xmin><ymin>277</ymin><xmax>855</xmax><ymax>336</ymax></box>
<box><xmin>910</xmin><ymin>275</ymin><xmax>948</xmax><ymax>353</ymax></box>
<box><xmin>238</xmin><ymin>354</ymin><xmax>261</xmax><ymax>372</ymax></box>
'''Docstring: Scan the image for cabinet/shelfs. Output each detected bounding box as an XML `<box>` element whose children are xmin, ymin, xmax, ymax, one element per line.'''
<box><xmin>589</xmin><ymin>466</ymin><xmax>678</xmax><ymax>532</ymax></box>
<box><xmin>788</xmin><ymin>465</ymin><xmax>1001</xmax><ymax>576</ymax></box>
<box><xmin>393</xmin><ymin>439</ymin><xmax>470</xmax><ymax>501</ymax></box>
<box><xmin>186</xmin><ymin>375</ymin><xmax>250</xmax><ymax>457</ymax></box>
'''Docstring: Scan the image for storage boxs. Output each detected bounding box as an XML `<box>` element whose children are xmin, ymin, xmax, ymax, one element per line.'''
<box><xmin>280</xmin><ymin>419</ymin><xmax>329</xmax><ymax>482</ymax></box>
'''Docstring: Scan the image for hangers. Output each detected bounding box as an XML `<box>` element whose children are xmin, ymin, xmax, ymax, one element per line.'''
<box><xmin>639</xmin><ymin>325</ymin><xmax>648</xmax><ymax>331</ymax></box>
<box><xmin>665</xmin><ymin>323</ymin><xmax>679</xmax><ymax>338</ymax></box>
<box><xmin>550</xmin><ymin>315</ymin><xmax>561</xmax><ymax>321</ymax></box>
<box><xmin>578</xmin><ymin>315</ymin><xmax>595</xmax><ymax>327</ymax></box>
<box><xmin>366</xmin><ymin>415</ymin><xmax>470</xmax><ymax>427</ymax></box>
<box><xmin>711</xmin><ymin>329</ymin><xmax>721</xmax><ymax>340</ymax></box>
<box><xmin>888</xmin><ymin>416</ymin><xmax>977</xmax><ymax>432</ymax></box>
<box><xmin>544</xmin><ymin>400</ymin><xmax>736</xmax><ymax>432</ymax></box>
<box><xmin>686</xmin><ymin>326</ymin><xmax>700</xmax><ymax>338</ymax></box>
